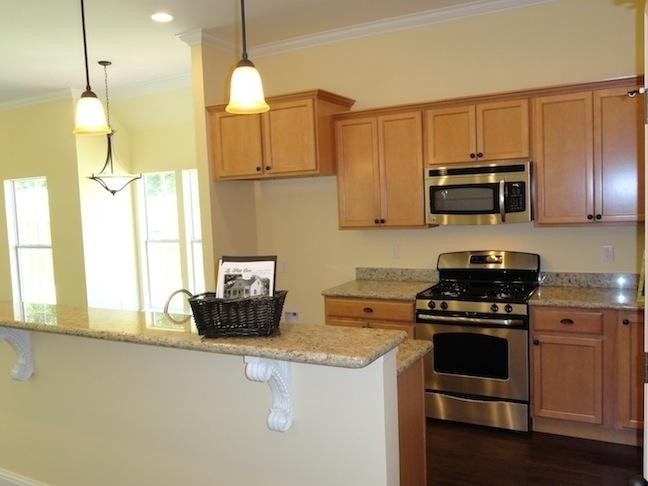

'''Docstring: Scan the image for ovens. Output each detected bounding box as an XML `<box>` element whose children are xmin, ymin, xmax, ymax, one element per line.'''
<box><xmin>414</xmin><ymin>310</ymin><xmax>532</xmax><ymax>432</ymax></box>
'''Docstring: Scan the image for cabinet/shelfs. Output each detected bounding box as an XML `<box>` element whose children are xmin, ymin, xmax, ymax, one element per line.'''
<box><xmin>423</xmin><ymin>98</ymin><xmax>530</xmax><ymax>165</ymax></box>
<box><xmin>616</xmin><ymin>311</ymin><xmax>644</xmax><ymax>431</ymax></box>
<box><xmin>204</xmin><ymin>88</ymin><xmax>355</xmax><ymax>181</ymax></box>
<box><xmin>534</xmin><ymin>85</ymin><xmax>645</xmax><ymax>224</ymax></box>
<box><xmin>324</xmin><ymin>296</ymin><xmax>416</xmax><ymax>340</ymax></box>
<box><xmin>336</xmin><ymin>110</ymin><xmax>440</xmax><ymax>227</ymax></box>
<box><xmin>532</xmin><ymin>306</ymin><xmax>603</xmax><ymax>425</ymax></box>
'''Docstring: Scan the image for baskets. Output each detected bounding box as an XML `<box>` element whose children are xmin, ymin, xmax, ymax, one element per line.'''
<box><xmin>188</xmin><ymin>290</ymin><xmax>289</xmax><ymax>337</ymax></box>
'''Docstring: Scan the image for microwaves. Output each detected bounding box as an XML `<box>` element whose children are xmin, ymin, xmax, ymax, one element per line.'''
<box><xmin>424</xmin><ymin>159</ymin><xmax>531</xmax><ymax>224</ymax></box>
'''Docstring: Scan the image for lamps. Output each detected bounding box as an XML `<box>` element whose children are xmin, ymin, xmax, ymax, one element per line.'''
<box><xmin>224</xmin><ymin>1</ymin><xmax>270</xmax><ymax>115</ymax></box>
<box><xmin>85</xmin><ymin>61</ymin><xmax>141</xmax><ymax>197</ymax></box>
<box><xmin>73</xmin><ymin>1</ymin><xmax>112</xmax><ymax>136</ymax></box>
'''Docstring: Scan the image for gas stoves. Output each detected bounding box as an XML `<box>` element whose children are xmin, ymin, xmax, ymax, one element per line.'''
<box><xmin>416</xmin><ymin>278</ymin><xmax>536</xmax><ymax>314</ymax></box>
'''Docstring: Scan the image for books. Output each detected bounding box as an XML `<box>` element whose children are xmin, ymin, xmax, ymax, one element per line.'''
<box><xmin>214</xmin><ymin>253</ymin><xmax>278</xmax><ymax>300</ymax></box>
